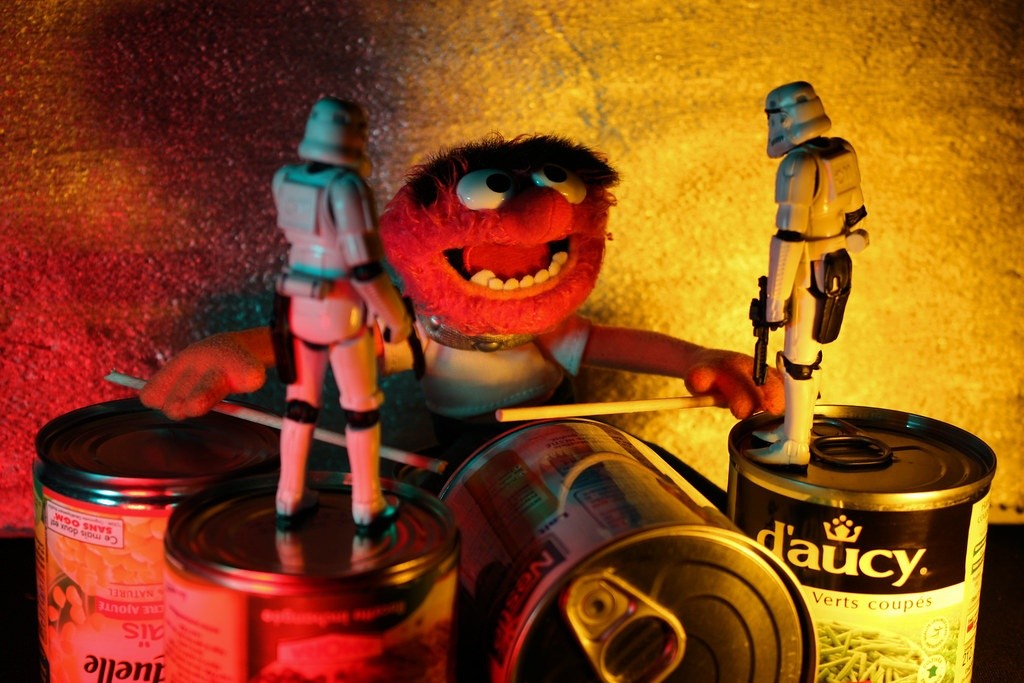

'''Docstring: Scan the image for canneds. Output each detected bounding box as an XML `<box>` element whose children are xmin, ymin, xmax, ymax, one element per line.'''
<box><xmin>33</xmin><ymin>396</ymin><xmax>998</xmax><ymax>683</ymax></box>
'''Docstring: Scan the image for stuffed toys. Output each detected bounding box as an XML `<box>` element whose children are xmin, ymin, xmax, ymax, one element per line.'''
<box><xmin>142</xmin><ymin>133</ymin><xmax>786</xmax><ymax>494</ymax></box>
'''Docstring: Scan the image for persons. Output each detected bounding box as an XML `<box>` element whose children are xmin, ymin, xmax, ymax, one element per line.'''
<box><xmin>746</xmin><ymin>79</ymin><xmax>870</xmax><ymax>470</ymax></box>
<box><xmin>270</xmin><ymin>97</ymin><xmax>413</xmax><ymax>535</ymax></box>
